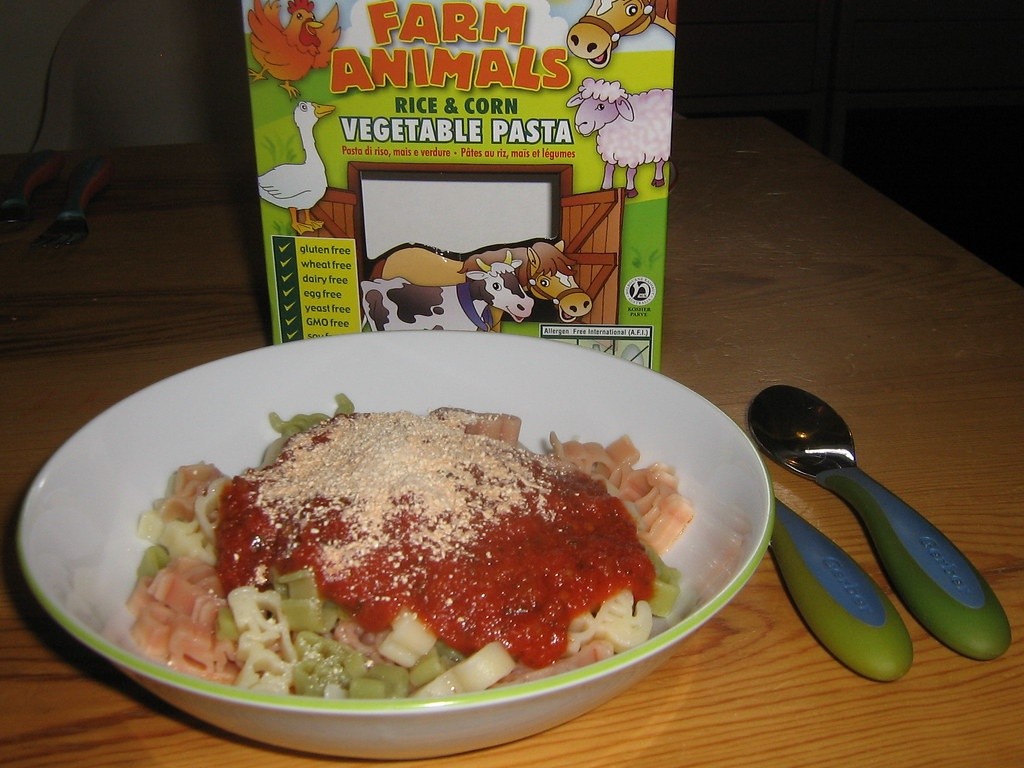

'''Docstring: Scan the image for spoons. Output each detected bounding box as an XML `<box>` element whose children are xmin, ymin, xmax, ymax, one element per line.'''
<box><xmin>748</xmin><ymin>384</ymin><xmax>1011</xmax><ymax>662</ymax></box>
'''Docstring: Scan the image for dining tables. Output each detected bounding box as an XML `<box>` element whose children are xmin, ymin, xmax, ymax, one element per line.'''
<box><xmin>0</xmin><ymin>114</ymin><xmax>1022</xmax><ymax>766</ymax></box>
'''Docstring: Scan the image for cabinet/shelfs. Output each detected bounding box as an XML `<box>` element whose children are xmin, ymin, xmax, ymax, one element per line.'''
<box><xmin>828</xmin><ymin>0</ymin><xmax>1022</xmax><ymax>160</ymax></box>
<box><xmin>678</xmin><ymin>0</ymin><xmax>838</xmax><ymax>155</ymax></box>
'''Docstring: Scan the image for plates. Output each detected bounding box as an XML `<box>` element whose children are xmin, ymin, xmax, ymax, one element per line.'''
<box><xmin>18</xmin><ymin>332</ymin><xmax>774</xmax><ymax>759</ymax></box>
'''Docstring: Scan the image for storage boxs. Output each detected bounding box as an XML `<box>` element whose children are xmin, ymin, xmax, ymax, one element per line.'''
<box><xmin>237</xmin><ymin>1</ymin><xmax>675</xmax><ymax>374</ymax></box>
